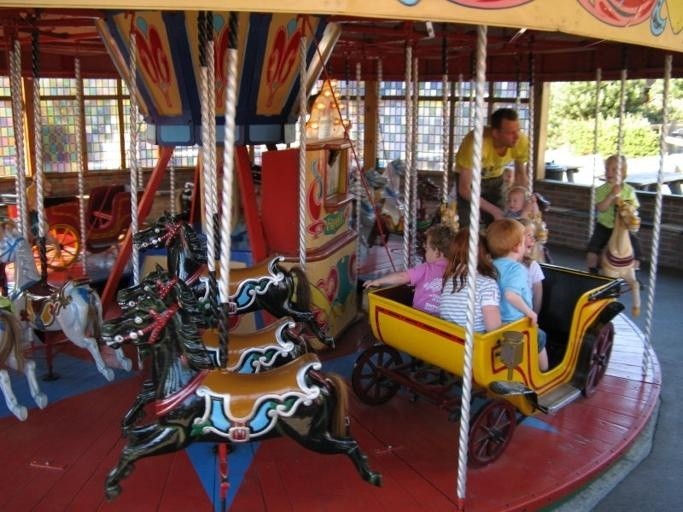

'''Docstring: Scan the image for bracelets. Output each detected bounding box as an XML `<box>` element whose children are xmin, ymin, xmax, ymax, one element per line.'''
<box><xmin>608</xmin><ymin>193</ymin><xmax>614</xmax><ymax>200</ymax></box>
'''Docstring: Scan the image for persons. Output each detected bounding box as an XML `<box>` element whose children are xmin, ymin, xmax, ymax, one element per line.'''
<box><xmin>585</xmin><ymin>153</ymin><xmax>647</xmax><ymax>292</ymax></box>
<box><xmin>363</xmin><ymin>223</ymin><xmax>454</xmax><ymax>318</ymax></box>
<box><xmin>518</xmin><ymin>217</ymin><xmax>546</xmax><ymax>314</ymax></box>
<box><xmin>506</xmin><ymin>186</ymin><xmax>554</xmax><ymax>265</ymax></box>
<box><xmin>485</xmin><ymin>218</ymin><xmax>538</xmax><ymax>325</ymax></box>
<box><xmin>26</xmin><ymin>171</ymin><xmax>45</xmax><ymax>235</ymax></box>
<box><xmin>454</xmin><ymin>108</ymin><xmax>529</xmax><ymax>228</ymax></box>
<box><xmin>503</xmin><ymin>168</ymin><xmax>514</xmax><ymax>189</ymax></box>
<box><xmin>444</xmin><ymin>229</ymin><xmax>549</xmax><ymax>373</ymax></box>
